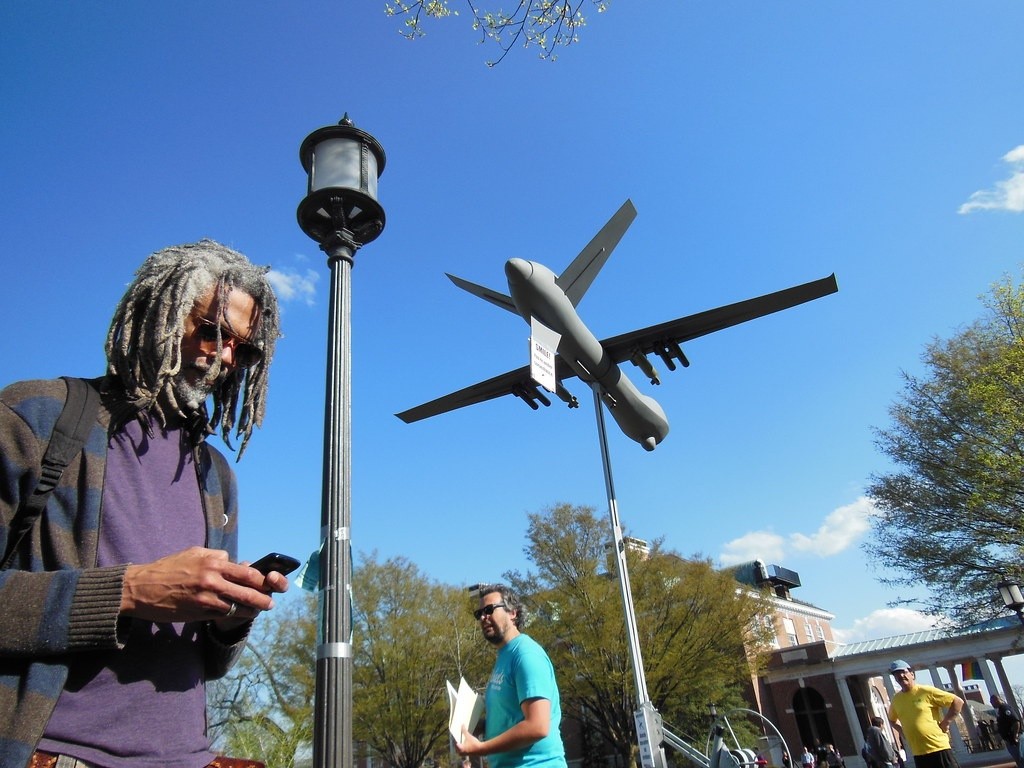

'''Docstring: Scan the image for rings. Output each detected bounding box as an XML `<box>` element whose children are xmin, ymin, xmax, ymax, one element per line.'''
<box><xmin>225</xmin><ymin>603</ymin><xmax>238</xmax><ymax>618</ymax></box>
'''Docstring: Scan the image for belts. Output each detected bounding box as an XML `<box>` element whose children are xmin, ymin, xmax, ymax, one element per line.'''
<box><xmin>29</xmin><ymin>751</ymin><xmax>266</xmax><ymax>768</ymax></box>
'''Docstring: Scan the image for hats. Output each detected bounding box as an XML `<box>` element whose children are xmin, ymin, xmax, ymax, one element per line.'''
<box><xmin>890</xmin><ymin>660</ymin><xmax>911</xmax><ymax>674</ymax></box>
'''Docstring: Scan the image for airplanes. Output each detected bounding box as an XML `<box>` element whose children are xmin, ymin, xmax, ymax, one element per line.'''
<box><xmin>396</xmin><ymin>197</ymin><xmax>840</xmax><ymax>451</ymax></box>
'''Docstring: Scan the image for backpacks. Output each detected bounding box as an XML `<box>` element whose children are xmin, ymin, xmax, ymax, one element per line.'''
<box><xmin>862</xmin><ymin>730</ymin><xmax>882</xmax><ymax>766</ymax></box>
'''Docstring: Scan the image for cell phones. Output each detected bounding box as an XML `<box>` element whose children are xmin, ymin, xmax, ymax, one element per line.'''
<box><xmin>248</xmin><ymin>553</ymin><xmax>301</xmax><ymax>577</ymax></box>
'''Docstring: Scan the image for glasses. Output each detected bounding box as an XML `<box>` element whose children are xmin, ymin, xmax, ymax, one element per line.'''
<box><xmin>474</xmin><ymin>603</ymin><xmax>505</xmax><ymax>620</ymax></box>
<box><xmin>188</xmin><ymin>312</ymin><xmax>265</xmax><ymax>367</ymax></box>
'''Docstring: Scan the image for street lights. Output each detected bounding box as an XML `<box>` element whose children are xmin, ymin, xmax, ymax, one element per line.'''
<box><xmin>295</xmin><ymin>112</ymin><xmax>386</xmax><ymax>768</ymax></box>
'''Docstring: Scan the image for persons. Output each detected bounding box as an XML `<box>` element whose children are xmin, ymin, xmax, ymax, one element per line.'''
<box><xmin>451</xmin><ymin>583</ymin><xmax>569</xmax><ymax>768</ymax></box>
<box><xmin>887</xmin><ymin>660</ymin><xmax>964</xmax><ymax>768</ymax></box>
<box><xmin>990</xmin><ymin>695</ymin><xmax>1024</xmax><ymax>768</ymax></box>
<box><xmin>1</xmin><ymin>237</ymin><xmax>289</xmax><ymax>768</ymax></box>
<box><xmin>977</xmin><ymin>713</ymin><xmax>1024</xmax><ymax>751</ymax></box>
<box><xmin>754</xmin><ymin>717</ymin><xmax>901</xmax><ymax>768</ymax></box>
